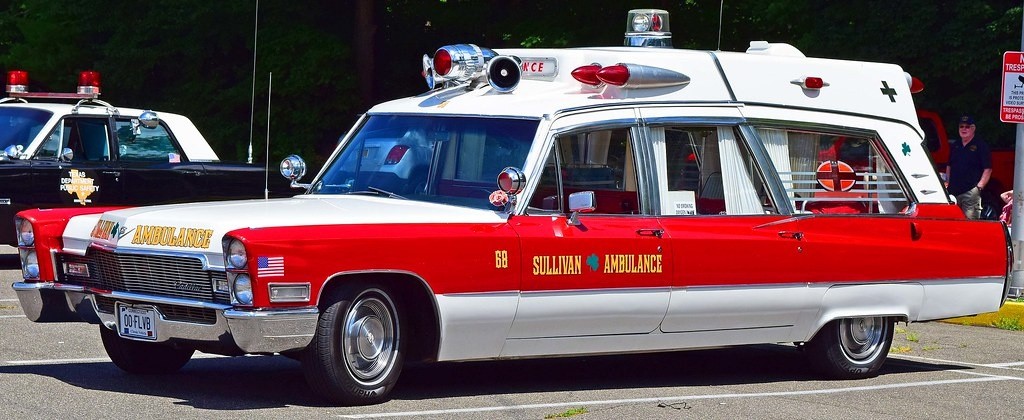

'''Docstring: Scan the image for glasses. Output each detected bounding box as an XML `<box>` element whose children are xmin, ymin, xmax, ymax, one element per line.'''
<box><xmin>959</xmin><ymin>125</ymin><xmax>970</xmax><ymax>129</ymax></box>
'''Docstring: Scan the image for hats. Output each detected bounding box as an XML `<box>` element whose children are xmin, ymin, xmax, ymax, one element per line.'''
<box><xmin>958</xmin><ymin>113</ymin><xmax>976</xmax><ymax>124</ymax></box>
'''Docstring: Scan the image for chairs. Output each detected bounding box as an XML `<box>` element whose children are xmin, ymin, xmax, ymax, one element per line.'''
<box><xmin>67</xmin><ymin>122</ymin><xmax>104</xmax><ymax>162</ymax></box>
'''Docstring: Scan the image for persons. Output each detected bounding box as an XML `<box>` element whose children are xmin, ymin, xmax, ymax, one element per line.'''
<box><xmin>946</xmin><ymin>115</ymin><xmax>992</xmax><ymax>220</ymax></box>
<box><xmin>999</xmin><ymin>189</ymin><xmax>1013</xmax><ymax>227</ymax></box>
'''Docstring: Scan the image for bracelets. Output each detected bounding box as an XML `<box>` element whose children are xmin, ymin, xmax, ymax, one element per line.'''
<box><xmin>976</xmin><ymin>185</ymin><xmax>982</xmax><ymax>191</ymax></box>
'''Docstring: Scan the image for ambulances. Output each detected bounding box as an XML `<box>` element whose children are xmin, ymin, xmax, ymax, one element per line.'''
<box><xmin>0</xmin><ymin>70</ymin><xmax>400</xmax><ymax>248</ymax></box>
<box><xmin>13</xmin><ymin>8</ymin><xmax>1016</xmax><ymax>407</ymax></box>
